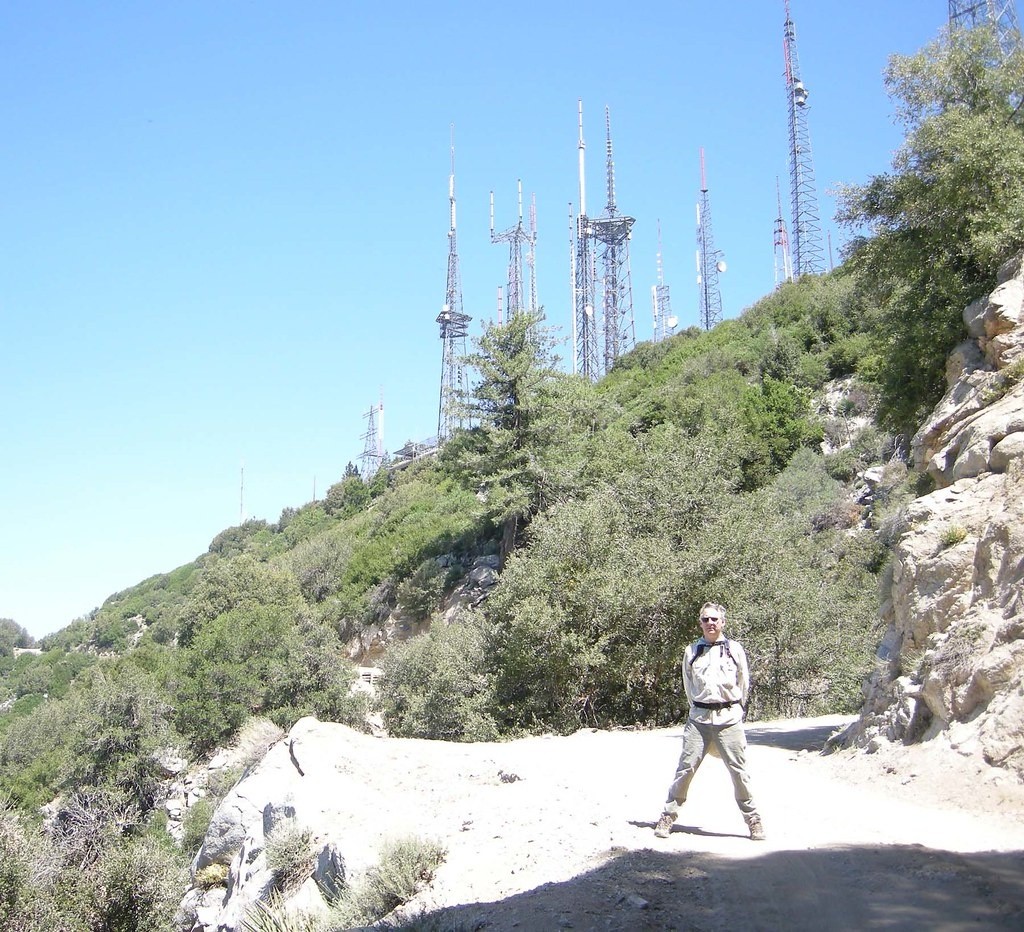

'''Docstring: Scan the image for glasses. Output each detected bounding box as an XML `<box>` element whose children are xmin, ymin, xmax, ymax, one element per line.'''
<box><xmin>701</xmin><ymin>617</ymin><xmax>722</xmax><ymax>623</ymax></box>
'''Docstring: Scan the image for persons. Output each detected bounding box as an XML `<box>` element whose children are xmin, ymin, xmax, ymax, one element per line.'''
<box><xmin>654</xmin><ymin>602</ymin><xmax>767</xmax><ymax>839</ymax></box>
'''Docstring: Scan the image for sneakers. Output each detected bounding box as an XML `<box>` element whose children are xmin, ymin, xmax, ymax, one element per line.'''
<box><xmin>654</xmin><ymin>815</ymin><xmax>676</xmax><ymax>838</ymax></box>
<box><xmin>749</xmin><ymin>823</ymin><xmax>765</xmax><ymax>840</ymax></box>
